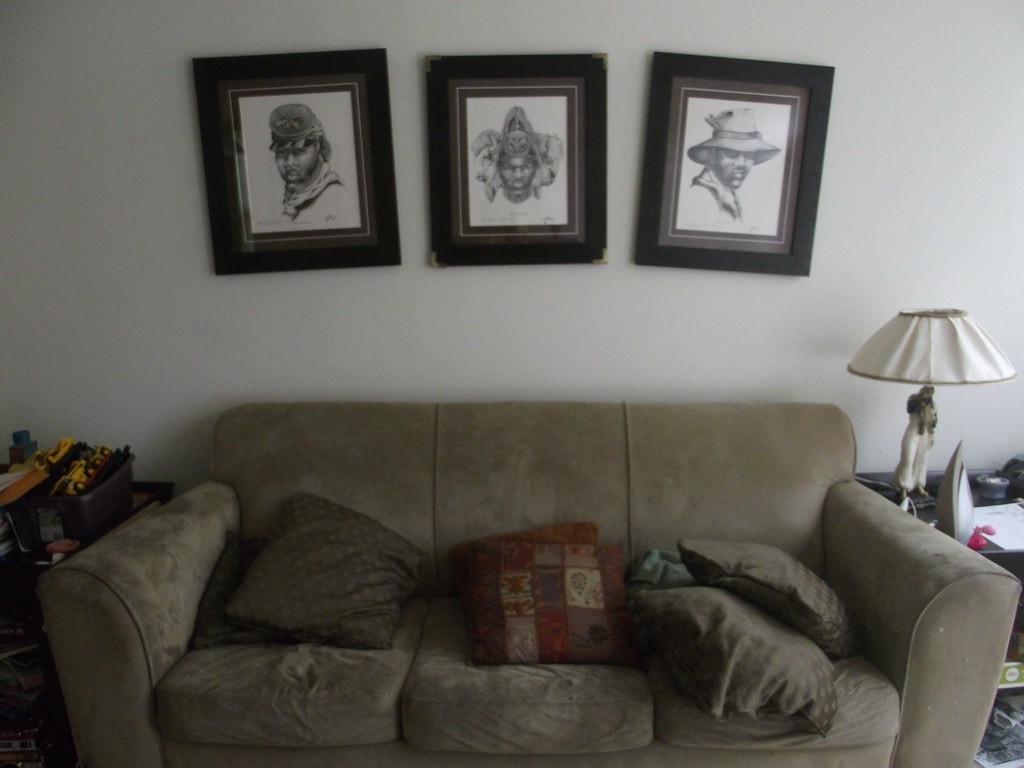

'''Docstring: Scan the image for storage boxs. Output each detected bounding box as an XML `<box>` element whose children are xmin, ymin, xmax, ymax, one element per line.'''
<box><xmin>21</xmin><ymin>451</ymin><xmax>136</xmax><ymax>548</ymax></box>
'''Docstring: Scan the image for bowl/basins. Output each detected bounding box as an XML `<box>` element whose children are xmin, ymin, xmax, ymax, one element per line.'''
<box><xmin>978</xmin><ymin>477</ymin><xmax>1009</xmax><ymax>499</ymax></box>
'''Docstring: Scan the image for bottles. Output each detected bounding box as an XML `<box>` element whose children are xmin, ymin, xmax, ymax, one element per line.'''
<box><xmin>9</xmin><ymin>429</ymin><xmax>37</xmax><ymax>466</ymax></box>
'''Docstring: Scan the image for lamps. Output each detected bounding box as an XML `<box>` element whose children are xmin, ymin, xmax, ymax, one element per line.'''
<box><xmin>847</xmin><ymin>308</ymin><xmax>1018</xmax><ymax>509</ymax></box>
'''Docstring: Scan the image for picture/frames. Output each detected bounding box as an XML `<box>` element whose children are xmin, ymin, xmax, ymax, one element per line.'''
<box><xmin>635</xmin><ymin>51</ymin><xmax>836</xmax><ymax>277</ymax></box>
<box><xmin>424</xmin><ymin>53</ymin><xmax>606</xmax><ymax>268</ymax></box>
<box><xmin>193</xmin><ymin>47</ymin><xmax>402</xmax><ymax>275</ymax></box>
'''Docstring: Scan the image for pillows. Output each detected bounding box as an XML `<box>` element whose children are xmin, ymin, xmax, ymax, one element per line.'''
<box><xmin>446</xmin><ymin>521</ymin><xmax>598</xmax><ymax>631</ymax></box>
<box><xmin>187</xmin><ymin>536</ymin><xmax>306</xmax><ymax>652</ymax></box>
<box><xmin>623</xmin><ymin>587</ymin><xmax>837</xmax><ymax>740</ymax></box>
<box><xmin>223</xmin><ymin>490</ymin><xmax>425</xmax><ymax>648</ymax></box>
<box><xmin>469</xmin><ymin>543</ymin><xmax>627</xmax><ymax>668</ymax></box>
<box><xmin>676</xmin><ymin>539</ymin><xmax>852</xmax><ymax>659</ymax></box>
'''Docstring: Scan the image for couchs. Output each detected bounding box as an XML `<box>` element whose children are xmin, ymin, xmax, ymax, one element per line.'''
<box><xmin>35</xmin><ymin>401</ymin><xmax>1023</xmax><ymax>768</ymax></box>
